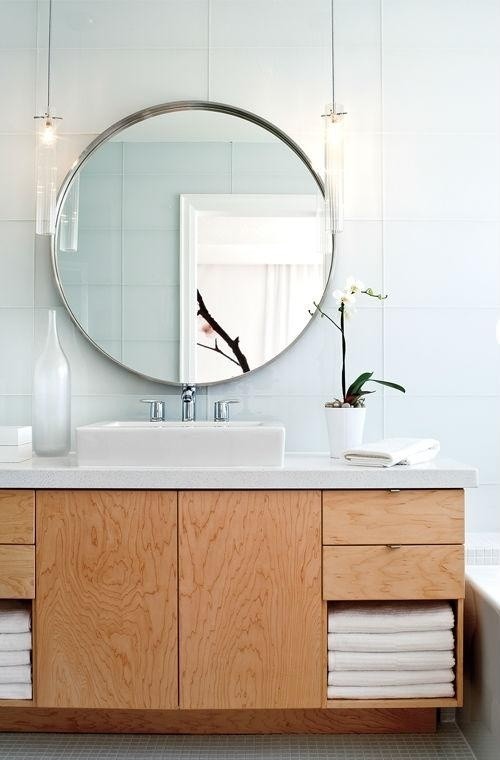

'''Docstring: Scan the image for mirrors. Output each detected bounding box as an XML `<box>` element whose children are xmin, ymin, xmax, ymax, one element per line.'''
<box><xmin>49</xmin><ymin>99</ymin><xmax>335</xmax><ymax>389</ymax></box>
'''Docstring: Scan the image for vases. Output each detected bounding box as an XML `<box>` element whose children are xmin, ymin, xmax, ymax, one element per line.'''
<box><xmin>325</xmin><ymin>409</ymin><xmax>366</xmax><ymax>459</ymax></box>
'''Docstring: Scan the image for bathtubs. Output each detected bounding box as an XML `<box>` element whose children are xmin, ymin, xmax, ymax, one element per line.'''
<box><xmin>457</xmin><ymin>563</ymin><xmax>500</xmax><ymax>760</ymax></box>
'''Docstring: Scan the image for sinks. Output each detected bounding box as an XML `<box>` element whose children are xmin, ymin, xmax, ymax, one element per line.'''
<box><xmin>75</xmin><ymin>422</ymin><xmax>285</xmax><ymax>464</ymax></box>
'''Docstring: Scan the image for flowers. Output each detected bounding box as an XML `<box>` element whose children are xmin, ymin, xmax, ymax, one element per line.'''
<box><xmin>310</xmin><ymin>282</ymin><xmax>405</xmax><ymax>408</ymax></box>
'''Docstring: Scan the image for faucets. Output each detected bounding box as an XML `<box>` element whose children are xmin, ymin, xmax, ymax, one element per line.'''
<box><xmin>181</xmin><ymin>387</ymin><xmax>197</xmax><ymax>421</ymax></box>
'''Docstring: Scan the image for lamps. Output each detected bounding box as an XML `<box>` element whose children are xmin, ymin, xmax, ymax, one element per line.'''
<box><xmin>59</xmin><ymin>173</ymin><xmax>80</xmax><ymax>252</ymax></box>
<box><xmin>318</xmin><ymin>0</ymin><xmax>349</xmax><ymax>233</ymax></box>
<box><xmin>32</xmin><ymin>0</ymin><xmax>59</xmax><ymax>236</ymax></box>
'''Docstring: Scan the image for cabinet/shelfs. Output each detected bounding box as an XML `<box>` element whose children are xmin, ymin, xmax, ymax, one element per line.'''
<box><xmin>0</xmin><ymin>489</ymin><xmax>466</xmax><ymax>736</ymax></box>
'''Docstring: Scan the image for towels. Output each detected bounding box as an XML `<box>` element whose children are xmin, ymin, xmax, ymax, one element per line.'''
<box><xmin>0</xmin><ymin>610</ymin><xmax>33</xmax><ymax>701</ymax></box>
<box><xmin>327</xmin><ymin>604</ymin><xmax>456</xmax><ymax>700</ymax></box>
<box><xmin>340</xmin><ymin>437</ymin><xmax>440</xmax><ymax>468</ymax></box>
